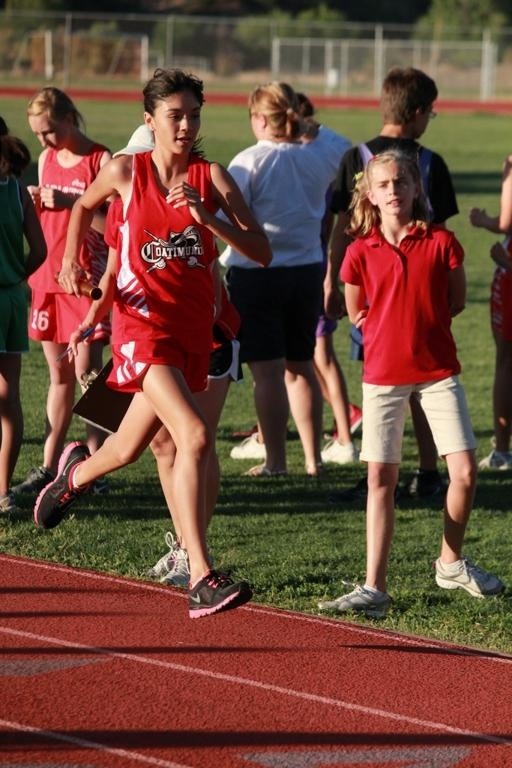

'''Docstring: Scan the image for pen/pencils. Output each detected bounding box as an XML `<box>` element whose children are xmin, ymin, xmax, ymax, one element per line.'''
<box><xmin>55</xmin><ymin>326</ymin><xmax>95</xmax><ymax>362</ymax></box>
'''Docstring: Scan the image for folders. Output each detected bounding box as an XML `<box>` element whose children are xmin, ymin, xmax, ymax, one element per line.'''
<box><xmin>72</xmin><ymin>356</ymin><xmax>134</xmax><ymax>434</ymax></box>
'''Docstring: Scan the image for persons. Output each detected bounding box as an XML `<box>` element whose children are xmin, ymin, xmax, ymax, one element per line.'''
<box><xmin>216</xmin><ymin>80</ymin><xmax>353</xmax><ymax>476</ymax></box>
<box><xmin>1</xmin><ymin>65</ymin><xmax>244</xmax><ymax>586</ymax></box>
<box><xmin>319</xmin><ymin>67</ymin><xmax>460</xmax><ymax>504</ymax></box>
<box><xmin>36</xmin><ymin>70</ymin><xmax>265</xmax><ymax>617</ymax></box>
<box><xmin>317</xmin><ymin>146</ymin><xmax>504</xmax><ymax>612</ymax></box>
<box><xmin>469</xmin><ymin>149</ymin><xmax>511</xmax><ymax>471</ymax></box>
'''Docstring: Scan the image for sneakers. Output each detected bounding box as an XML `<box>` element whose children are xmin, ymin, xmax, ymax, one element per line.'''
<box><xmin>231</xmin><ymin>426</ymin><xmax>287</xmax><ymax>476</ymax></box>
<box><xmin>0</xmin><ymin>441</ymin><xmax>107</xmax><ymax>529</ymax></box>
<box><xmin>151</xmin><ymin>532</ymin><xmax>253</xmax><ymax>618</ymax></box>
<box><xmin>401</xmin><ymin>469</ymin><xmax>442</xmax><ymax>498</ymax></box>
<box><xmin>478</xmin><ymin>449</ymin><xmax>512</xmax><ymax>471</ymax></box>
<box><xmin>320</xmin><ymin>402</ymin><xmax>362</xmax><ymax>464</ymax></box>
<box><xmin>435</xmin><ymin>558</ymin><xmax>505</xmax><ymax>598</ymax></box>
<box><xmin>318</xmin><ymin>581</ymin><xmax>392</xmax><ymax>620</ymax></box>
